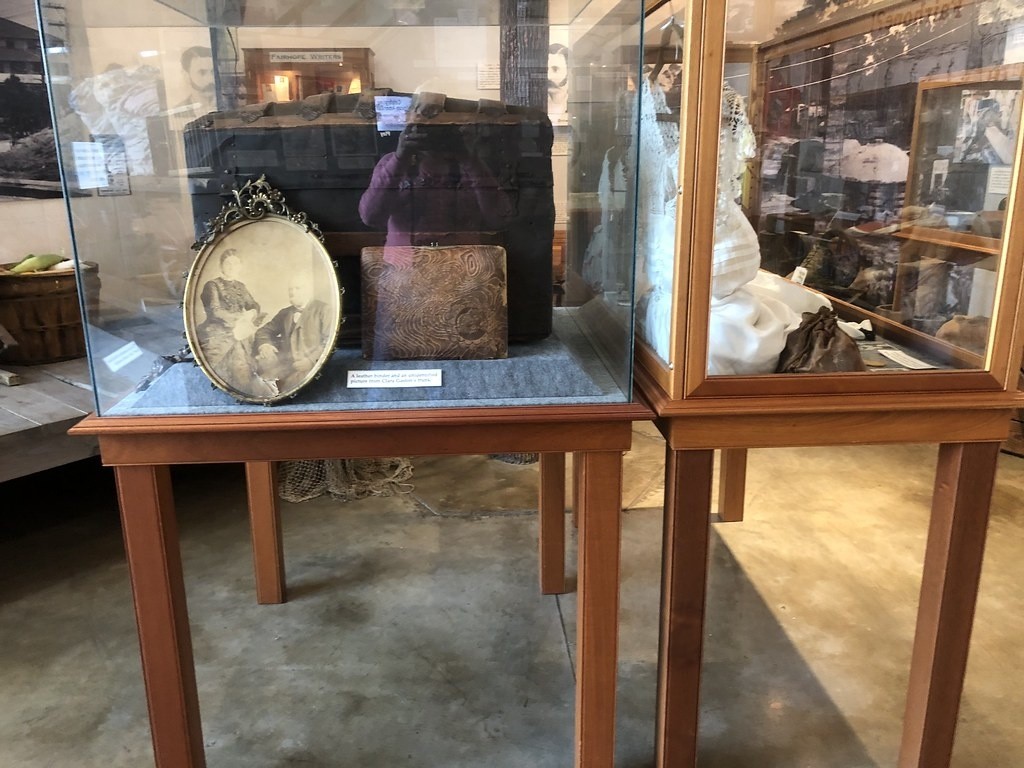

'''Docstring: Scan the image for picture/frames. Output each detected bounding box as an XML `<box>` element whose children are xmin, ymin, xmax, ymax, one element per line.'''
<box><xmin>183</xmin><ymin>173</ymin><xmax>346</xmax><ymax>403</ymax></box>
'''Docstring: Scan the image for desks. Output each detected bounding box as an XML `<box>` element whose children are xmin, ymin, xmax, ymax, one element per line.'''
<box><xmin>0</xmin><ymin>306</ymin><xmax>192</xmax><ymax>482</ymax></box>
<box><xmin>67</xmin><ymin>306</ymin><xmax>1024</xmax><ymax>767</ymax></box>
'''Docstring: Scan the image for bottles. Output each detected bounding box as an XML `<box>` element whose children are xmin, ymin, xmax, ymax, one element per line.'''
<box><xmin>929</xmin><ymin>174</ymin><xmax>943</xmax><ymax>204</ymax></box>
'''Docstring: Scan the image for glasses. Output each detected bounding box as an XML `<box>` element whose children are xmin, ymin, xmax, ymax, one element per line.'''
<box><xmin>420</xmin><ymin>103</ymin><xmax>442</xmax><ymax>119</ymax></box>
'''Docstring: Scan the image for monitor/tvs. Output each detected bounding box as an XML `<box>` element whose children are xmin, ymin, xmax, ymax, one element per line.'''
<box><xmin>372</xmin><ymin>94</ymin><xmax>413</xmax><ymax>132</ymax></box>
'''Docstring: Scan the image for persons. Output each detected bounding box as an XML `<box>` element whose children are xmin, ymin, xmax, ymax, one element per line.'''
<box><xmin>196</xmin><ymin>248</ymin><xmax>331</xmax><ymax>399</ymax></box>
<box><xmin>360</xmin><ymin>81</ymin><xmax>511</xmax><ymax>406</ymax></box>
<box><xmin>546</xmin><ymin>44</ymin><xmax>571</xmax><ymax>114</ymax></box>
<box><xmin>169</xmin><ymin>45</ymin><xmax>215</xmax><ymax>118</ymax></box>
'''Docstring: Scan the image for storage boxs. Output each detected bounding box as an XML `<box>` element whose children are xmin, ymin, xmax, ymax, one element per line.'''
<box><xmin>183</xmin><ymin>88</ymin><xmax>555</xmax><ymax>350</ymax></box>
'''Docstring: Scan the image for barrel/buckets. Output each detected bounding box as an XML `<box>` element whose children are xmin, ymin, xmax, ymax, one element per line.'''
<box><xmin>945</xmin><ymin>163</ymin><xmax>989</xmax><ymax>212</ymax></box>
<box><xmin>0</xmin><ymin>261</ymin><xmax>101</xmax><ymax>369</ymax></box>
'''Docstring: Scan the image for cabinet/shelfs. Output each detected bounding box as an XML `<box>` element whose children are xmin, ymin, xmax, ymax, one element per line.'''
<box><xmin>749</xmin><ymin>0</ymin><xmax>1024</xmax><ymax>455</ymax></box>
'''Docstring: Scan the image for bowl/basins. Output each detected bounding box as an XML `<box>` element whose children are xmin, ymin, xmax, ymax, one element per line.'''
<box><xmin>913</xmin><ymin>315</ymin><xmax>946</xmax><ymax>336</ymax></box>
<box><xmin>944</xmin><ymin>212</ymin><xmax>978</xmax><ymax>230</ymax></box>
